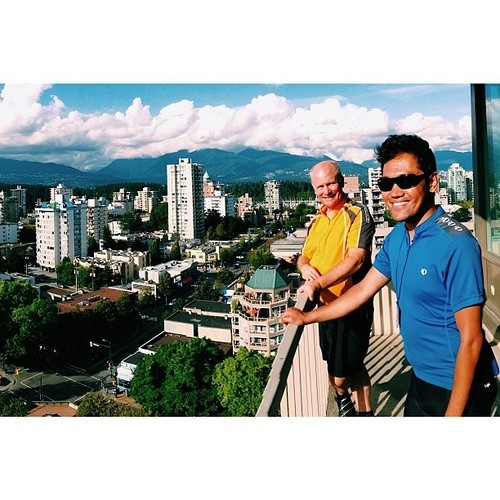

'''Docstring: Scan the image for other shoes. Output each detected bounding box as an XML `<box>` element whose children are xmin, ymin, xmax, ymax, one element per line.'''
<box><xmin>336</xmin><ymin>389</ymin><xmax>356</xmax><ymax>417</ymax></box>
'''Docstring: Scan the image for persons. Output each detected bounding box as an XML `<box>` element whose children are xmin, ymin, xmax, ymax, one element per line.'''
<box><xmin>240</xmin><ymin>290</ymin><xmax>259</xmax><ymax>321</ymax></box>
<box><xmin>295</xmin><ymin>160</ymin><xmax>378</xmax><ymax>417</ymax></box>
<box><xmin>277</xmin><ymin>132</ymin><xmax>500</xmax><ymax>417</ymax></box>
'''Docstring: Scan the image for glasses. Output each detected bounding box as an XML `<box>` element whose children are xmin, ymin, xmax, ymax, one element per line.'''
<box><xmin>377</xmin><ymin>173</ymin><xmax>430</xmax><ymax>192</ymax></box>
